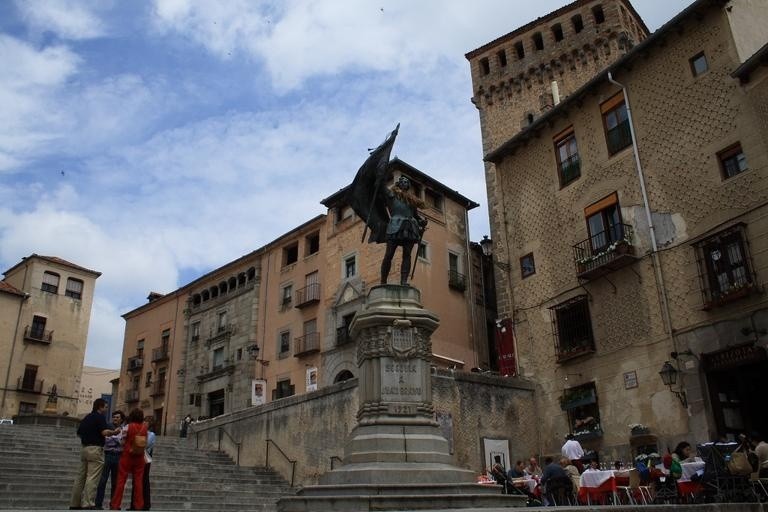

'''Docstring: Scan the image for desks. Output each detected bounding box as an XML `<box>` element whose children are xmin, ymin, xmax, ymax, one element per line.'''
<box><xmin>513</xmin><ymin>476</ymin><xmax>544</xmax><ymax>506</ymax></box>
<box><xmin>546</xmin><ymin>456</ymin><xmax>768</xmax><ymax>506</ymax></box>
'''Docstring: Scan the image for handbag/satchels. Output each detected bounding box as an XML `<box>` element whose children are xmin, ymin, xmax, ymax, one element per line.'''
<box><xmin>728</xmin><ymin>453</ymin><xmax>753</xmax><ymax>476</ymax></box>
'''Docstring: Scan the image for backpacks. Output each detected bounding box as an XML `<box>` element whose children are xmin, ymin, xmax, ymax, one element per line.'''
<box><xmin>133</xmin><ymin>423</ymin><xmax>145</xmax><ymax>447</ymax></box>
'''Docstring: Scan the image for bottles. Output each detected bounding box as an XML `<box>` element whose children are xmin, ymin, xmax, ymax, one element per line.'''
<box><xmin>599</xmin><ymin>462</ymin><xmax>632</xmax><ymax>471</ymax></box>
<box><xmin>523</xmin><ymin>470</ymin><xmax>527</xmax><ymax>480</ymax></box>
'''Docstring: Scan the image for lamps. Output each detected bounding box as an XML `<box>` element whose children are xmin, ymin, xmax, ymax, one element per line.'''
<box><xmin>252</xmin><ymin>345</ymin><xmax>269</xmax><ymax>366</ymax></box>
<box><xmin>658</xmin><ymin>361</ymin><xmax>688</xmax><ymax>409</ymax></box>
<box><xmin>480</xmin><ymin>236</ymin><xmax>496</xmax><ymax>264</ymax></box>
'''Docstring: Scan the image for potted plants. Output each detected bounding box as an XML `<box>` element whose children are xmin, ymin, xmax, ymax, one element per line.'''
<box><xmin>615</xmin><ymin>240</ymin><xmax>629</xmax><ymax>255</ymax></box>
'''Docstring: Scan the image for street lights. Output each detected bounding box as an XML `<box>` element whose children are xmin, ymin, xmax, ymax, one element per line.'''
<box><xmin>126</xmin><ymin>370</ymin><xmax>133</xmax><ymax>417</ymax></box>
<box><xmin>151</xmin><ymin>359</ymin><xmax>158</xmax><ymax>422</ymax></box>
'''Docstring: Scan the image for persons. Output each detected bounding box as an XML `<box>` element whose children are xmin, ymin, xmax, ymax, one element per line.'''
<box><xmin>381</xmin><ymin>176</ymin><xmax>428</xmax><ymax>285</ymax></box>
<box><xmin>492</xmin><ymin>432</ymin><xmax>768</xmax><ymax>506</ymax></box>
<box><xmin>69</xmin><ymin>398</ymin><xmax>161</xmax><ymax>511</ymax></box>
<box><xmin>180</xmin><ymin>414</ymin><xmax>211</xmax><ymax>437</ymax></box>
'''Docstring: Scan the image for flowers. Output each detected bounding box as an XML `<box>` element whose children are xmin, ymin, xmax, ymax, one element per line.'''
<box><xmin>628</xmin><ymin>424</ymin><xmax>649</xmax><ymax>435</ymax></box>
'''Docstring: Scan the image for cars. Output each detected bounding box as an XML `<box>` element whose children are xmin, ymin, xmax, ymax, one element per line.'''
<box><xmin>1</xmin><ymin>419</ymin><xmax>15</xmax><ymax>425</ymax></box>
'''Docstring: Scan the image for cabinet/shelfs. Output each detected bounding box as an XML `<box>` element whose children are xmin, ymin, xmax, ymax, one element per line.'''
<box><xmin>630</xmin><ymin>434</ymin><xmax>658</xmax><ymax>467</ymax></box>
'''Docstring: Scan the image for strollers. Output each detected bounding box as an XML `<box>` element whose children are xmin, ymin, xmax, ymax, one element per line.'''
<box><xmin>636</xmin><ymin>458</ymin><xmax>682</xmax><ymax>504</ymax></box>
<box><xmin>698</xmin><ymin>429</ymin><xmax>759</xmax><ymax>501</ymax></box>
